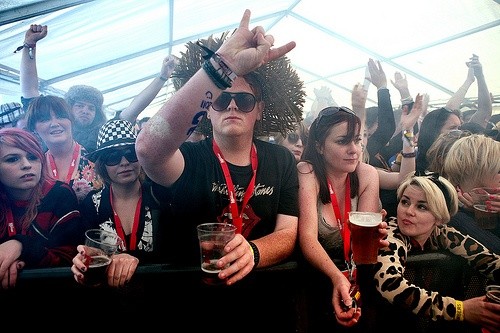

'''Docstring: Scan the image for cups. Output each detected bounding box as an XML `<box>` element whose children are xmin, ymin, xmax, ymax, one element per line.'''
<box><xmin>348</xmin><ymin>211</ymin><xmax>382</xmax><ymax>265</ymax></box>
<box><xmin>77</xmin><ymin>229</ymin><xmax>123</xmax><ymax>288</ymax></box>
<box><xmin>480</xmin><ymin>283</ymin><xmax>500</xmax><ymax>333</ymax></box>
<box><xmin>197</xmin><ymin>222</ymin><xmax>238</xmax><ymax>285</ymax></box>
<box><xmin>471</xmin><ymin>188</ymin><xmax>499</xmax><ymax>231</ymax></box>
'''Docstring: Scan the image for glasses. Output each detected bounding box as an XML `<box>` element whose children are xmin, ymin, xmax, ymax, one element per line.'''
<box><xmin>434</xmin><ymin>106</ymin><xmax>462</xmax><ymax>126</ymax></box>
<box><xmin>315</xmin><ymin>105</ymin><xmax>359</xmax><ymax>130</ymax></box>
<box><xmin>211</xmin><ymin>91</ymin><xmax>259</xmax><ymax>112</ymax></box>
<box><xmin>100</xmin><ymin>148</ymin><xmax>140</xmax><ymax>166</ymax></box>
<box><xmin>287</xmin><ymin>131</ymin><xmax>308</xmax><ymax>145</ymax></box>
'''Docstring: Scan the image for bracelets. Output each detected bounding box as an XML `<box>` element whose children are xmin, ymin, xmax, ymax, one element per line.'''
<box><xmin>156</xmin><ymin>72</ymin><xmax>169</xmax><ymax>81</ymax></box>
<box><xmin>247</xmin><ymin>241</ymin><xmax>259</xmax><ymax>268</ymax></box>
<box><xmin>455</xmin><ymin>300</ymin><xmax>463</xmax><ymax>320</ymax></box>
<box><xmin>196</xmin><ymin>41</ymin><xmax>236</xmax><ymax>90</ymax></box>
<box><xmin>14</xmin><ymin>41</ymin><xmax>37</xmax><ymax>60</ymax></box>
<box><xmin>401</xmin><ymin>150</ymin><xmax>416</xmax><ymax>157</ymax></box>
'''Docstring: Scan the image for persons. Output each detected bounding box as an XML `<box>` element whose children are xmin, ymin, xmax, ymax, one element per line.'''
<box><xmin>71</xmin><ymin>120</ymin><xmax>159</xmax><ymax>287</ymax></box>
<box><xmin>28</xmin><ymin>96</ymin><xmax>102</xmax><ymax>201</ymax></box>
<box><xmin>136</xmin><ymin>9</ymin><xmax>299</xmax><ymax>284</ymax></box>
<box><xmin>14</xmin><ymin>25</ymin><xmax>176</xmax><ymax>153</ymax></box>
<box><xmin>294</xmin><ymin>106</ymin><xmax>389</xmax><ymax>325</ymax></box>
<box><xmin>351</xmin><ymin>172</ymin><xmax>500</xmax><ymax>333</ymax></box>
<box><xmin>0</xmin><ymin>128</ymin><xmax>81</xmax><ymax>288</ymax></box>
<box><xmin>139</xmin><ymin>117</ymin><xmax>150</xmax><ymax>128</ymax></box>
<box><xmin>271</xmin><ymin>53</ymin><xmax>500</xmax><ymax>212</ymax></box>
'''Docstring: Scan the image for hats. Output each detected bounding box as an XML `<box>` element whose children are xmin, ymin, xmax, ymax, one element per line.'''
<box><xmin>85</xmin><ymin>118</ymin><xmax>139</xmax><ymax>164</ymax></box>
<box><xmin>170</xmin><ymin>28</ymin><xmax>305</xmax><ymax>138</ymax></box>
<box><xmin>64</xmin><ymin>84</ymin><xmax>106</xmax><ymax>124</ymax></box>
